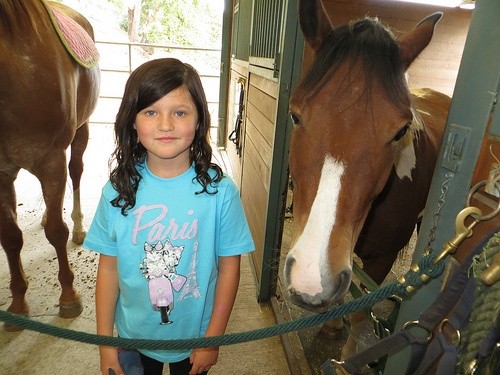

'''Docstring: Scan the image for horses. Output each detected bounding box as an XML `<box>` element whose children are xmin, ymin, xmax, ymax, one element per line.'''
<box><xmin>0</xmin><ymin>0</ymin><xmax>101</xmax><ymax>333</ymax></box>
<box><xmin>263</xmin><ymin>0</ymin><xmax>452</xmax><ymax>375</ymax></box>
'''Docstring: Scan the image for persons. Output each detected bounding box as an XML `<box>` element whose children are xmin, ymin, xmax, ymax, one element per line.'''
<box><xmin>83</xmin><ymin>57</ymin><xmax>257</xmax><ymax>375</ymax></box>
